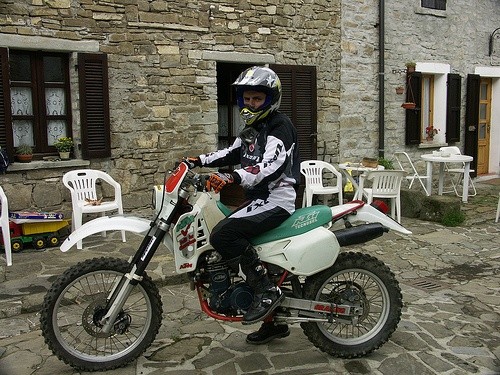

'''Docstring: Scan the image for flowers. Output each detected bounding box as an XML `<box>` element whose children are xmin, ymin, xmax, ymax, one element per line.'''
<box><xmin>54</xmin><ymin>138</ymin><xmax>71</xmax><ymax>152</ymax></box>
<box><xmin>425</xmin><ymin>125</ymin><xmax>437</xmax><ymax>137</ymax></box>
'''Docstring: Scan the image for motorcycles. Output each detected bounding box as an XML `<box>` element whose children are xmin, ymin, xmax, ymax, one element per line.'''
<box><xmin>39</xmin><ymin>155</ymin><xmax>403</xmax><ymax>372</ymax></box>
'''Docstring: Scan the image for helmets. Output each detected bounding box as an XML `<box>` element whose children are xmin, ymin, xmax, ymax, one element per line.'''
<box><xmin>232</xmin><ymin>67</ymin><xmax>282</xmax><ymax>126</ymax></box>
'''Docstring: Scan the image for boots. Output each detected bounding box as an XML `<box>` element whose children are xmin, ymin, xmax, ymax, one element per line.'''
<box><xmin>244</xmin><ymin>323</ymin><xmax>290</xmax><ymax>345</ymax></box>
<box><xmin>224</xmin><ymin>244</ymin><xmax>286</xmax><ymax>325</ymax></box>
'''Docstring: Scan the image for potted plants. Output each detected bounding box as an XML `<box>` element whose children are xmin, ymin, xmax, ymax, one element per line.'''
<box><xmin>396</xmin><ymin>85</ymin><xmax>405</xmax><ymax>93</ymax></box>
<box><xmin>17</xmin><ymin>144</ymin><xmax>33</xmax><ymax>161</ymax></box>
<box><xmin>402</xmin><ymin>102</ymin><xmax>417</xmax><ymax>108</ymax></box>
<box><xmin>405</xmin><ymin>61</ymin><xmax>416</xmax><ymax>72</ymax></box>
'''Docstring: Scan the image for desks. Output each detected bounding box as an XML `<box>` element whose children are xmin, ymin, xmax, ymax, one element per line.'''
<box><xmin>339</xmin><ymin>163</ymin><xmax>384</xmax><ymax>202</ymax></box>
<box><xmin>420</xmin><ymin>155</ymin><xmax>474</xmax><ymax>204</ymax></box>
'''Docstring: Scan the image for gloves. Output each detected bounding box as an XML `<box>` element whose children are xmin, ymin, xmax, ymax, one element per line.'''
<box><xmin>206</xmin><ymin>172</ymin><xmax>235</xmax><ymax>193</ymax></box>
<box><xmin>173</xmin><ymin>156</ymin><xmax>199</xmax><ymax>170</ymax></box>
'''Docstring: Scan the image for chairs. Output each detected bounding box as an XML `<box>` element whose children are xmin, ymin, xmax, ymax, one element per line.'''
<box><xmin>61</xmin><ymin>168</ymin><xmax>126</xmax><ymax>250</ymax></box>
<box><xmin>356</xmin><ymin>147</ymin><xmax>432</xmax><ymax>224</ymax></box>
<box><xmin>300</xmin><ymin>160</ymin><xmax>344</xmax><ymax>206</ymax></box>
<box><xmin>439</xmin><ymin>147</ymin><xmax>477</xmax><ymax>199</ymax></box>
<box><xmin>0</xmin><ymin>186</ymin><xmax>12</xmax><ymax>266</ymax></box>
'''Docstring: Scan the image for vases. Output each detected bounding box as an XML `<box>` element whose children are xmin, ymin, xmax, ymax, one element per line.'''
<box><xmin>60</xmin><ymin>150</ymin><xmax>69</xmax><ymax>158</ymax></box>
<box><xmin>426</xmin><ymin>136</ymin><xmax>434</xmax><ymax>141</ymax></box>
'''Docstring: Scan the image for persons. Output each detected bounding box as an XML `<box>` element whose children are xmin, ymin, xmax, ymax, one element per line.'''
<box><xmin>172</xmin><ymin>66</ymin><xmax>300</xmax><ymax>345</ymax></box>
